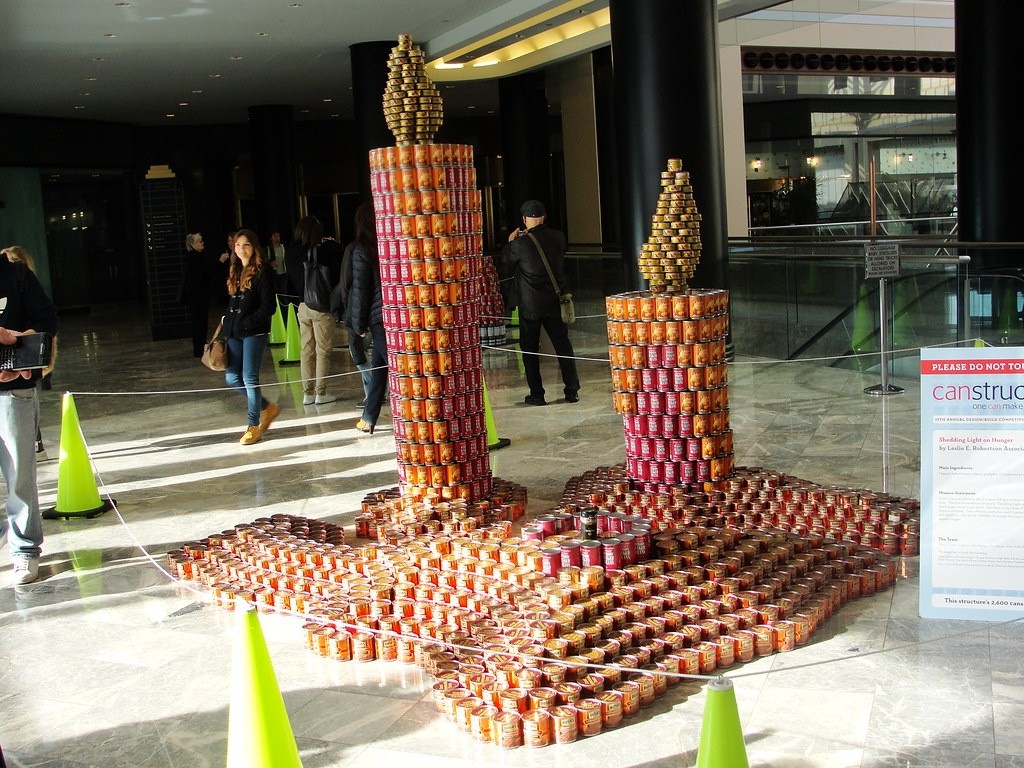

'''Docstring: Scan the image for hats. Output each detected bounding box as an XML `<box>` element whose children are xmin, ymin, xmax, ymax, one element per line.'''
<box><xmin>519</xmin><ymin>200</ymin><xmax>546</xmax><ymax>218</ymax></box>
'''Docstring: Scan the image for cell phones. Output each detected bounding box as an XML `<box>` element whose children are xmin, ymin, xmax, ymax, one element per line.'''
<box><xmin>0</xmin><ymin>332</ymin><xmax>53</xmax><ymax>371</ymax></box>
<box><xmin>519</xmin><ymin>231</ymin><xmax>525</xmax><ymax>236</ymax></box>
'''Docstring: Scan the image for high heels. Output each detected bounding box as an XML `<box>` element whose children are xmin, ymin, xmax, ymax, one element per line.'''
<box><xmin>355</xmin><ymin>418</ymin><xmax>374</xmax><ymax>434</ymax></box>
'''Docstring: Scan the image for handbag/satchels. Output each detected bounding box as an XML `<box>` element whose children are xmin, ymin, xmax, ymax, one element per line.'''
<box><xmin>302</xmin><ymin>246</ymin><xmax>341</xmax><ymax>313</ymax></box>
<box><xmin>200</xmin><ymin>316</ymin><xmax>228</xmax><ymax>372</ymax></box>
<box><xmin>558</xmin><ymin>293</ymin><xmax>576</xmax><ymax>324</ymax></box>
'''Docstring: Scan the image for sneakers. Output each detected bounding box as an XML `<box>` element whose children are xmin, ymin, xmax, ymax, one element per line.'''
<box><xmin>0</xmin><ymin>530</ymin><xmax>9</xmax><ymax>551</ymax></box>
<box><xmin>12</xmin><ymin>557</ymin><xmax>40</xmax><ymax>585</ymax></box>
<box><xmin>34</xmin><ymin>440</ymin><xmax>49</xmax><ymax>463</ymax></box>
<box><xmin>315</xmin><ymin>392</ymin><xmax>337</xmax><ymax>405</ymax></box>
<box><xmin>302</xmin><ymin>393</ymin><xmax>315</xmax><ymax>405</ymax></box>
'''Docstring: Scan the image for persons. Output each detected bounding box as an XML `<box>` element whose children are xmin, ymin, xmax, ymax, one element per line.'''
<box><xmin>264</xmin><ymin>231</ymin><xmax>288</xmax><ymax>295</ymax></box>
<box><xmin>186</xmin><ymin>233</ymin><xmax>229</xmax><ymax>357</ymax></box>
<box><xmin>0</xmin><ymin>246</ymin><xmax>57</xmax><ymax>462</ymax></box>
<box><xmin>502</xmin><ymin>200</ymin><xmax>581</xmax><ymax>405</ymax></box>
<box><xmin>0</xmin><ymin>254</ymin><xmax>58</xmax><ymax>585</ymax></box>
<box><xmin>284</xmin><ymin>216</ymin><xmax>345</xmax><ymax>405</ymax></box>
<box><xmin>227</xmin><ymin>232</ymin><xmax>237</xmax><ymax>251</ymax></box>
<box><xmin>225</xmin><ymin>229</ymin><xmax>281</xmax><ymax>445</ymax></box>
<box><xmin>0</xmin><ymin>326</ymin><xmax>37</xmax><ymax>382</ymax></box>
<box><xmin>349</xmin><ymin>328</ymin><xmax>387</xmax><ymax>408</ymax></box>
<box><xmin>339</xmin><ymin>202</ymin><xmax>388</xmax><ymax>434</ymax></box>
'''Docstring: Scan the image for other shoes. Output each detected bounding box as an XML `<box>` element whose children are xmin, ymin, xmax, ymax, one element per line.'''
<box><xmin>194</xmin><ymin>354</ymin><xmax>204</xmax><ymax>361</ymax></box>
<box><xmin>356</xmin><ymin>401</ymin><xmax>366</xmax><ymax>408</ymax></box>
<box><xmin>525</xmin><ymin>395</ymin><xmax>547</xmax><ymax>406</ymax></box>
<box><xmin>381</xmin><ymin>400</ymin><xmax>388</xmax><ymax>406</ymax></box>
<box><xmin>565</xmin><ymin>393</ymin><xmax>580</xmax><ymax>402</ymax></box>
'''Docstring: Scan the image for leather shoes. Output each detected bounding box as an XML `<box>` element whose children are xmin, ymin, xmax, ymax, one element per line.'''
<box><xmin>239</xmin><ymin>426</ymin><xmax>262</xmax><ymax>445</ymax></box>
<box><xmin>258</xmin><ymin>404</ymin><xmax>281</xmax><ymax>434</ymax></box>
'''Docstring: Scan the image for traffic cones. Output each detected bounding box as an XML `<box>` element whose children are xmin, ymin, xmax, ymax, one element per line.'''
<box><xmin>845</xmin><ymin>277</ymin><xmax>913</xmax><ymax>352</ymax></box>
<box><xmin>269</xmin><ymin>346</ymin><xmax>287</xmax><ymax>394</ymax></box>
<box><xmin>226</xmin><ymin>605</ymin><xmax>303</xmax><ymax>768</ymax></box>
<box><xmin>58</xmin><ymin>517</ymin><xmax>104</xmax><ymax>611</ymax></box>
<box><xmin>268</xmin><ymin>294</ymin><xmax>287</xmax><ymax>345</ymax></box>
<box><xmin>279</xmin><ymin>365</ymin><xmax>304</xmax><ymax>416</ymax></box>
<box><xmin>278</xmin><ymin>303</ymin><xmax>301</xmax><ymax>364</ymax></box>
<box><xmin>43</xmin><ymin>393</ymin><xmax>117</xmax><ymax>519</ymax></box>
<box><xmin>696</xmin><ymin>677</ymin><xmax>749</xmax><ymax>768</ymax></box>
<box><xmin>484</xmin><ymin>374</ymin><xmax>511</xmax><ymax>452</ymax></box>
